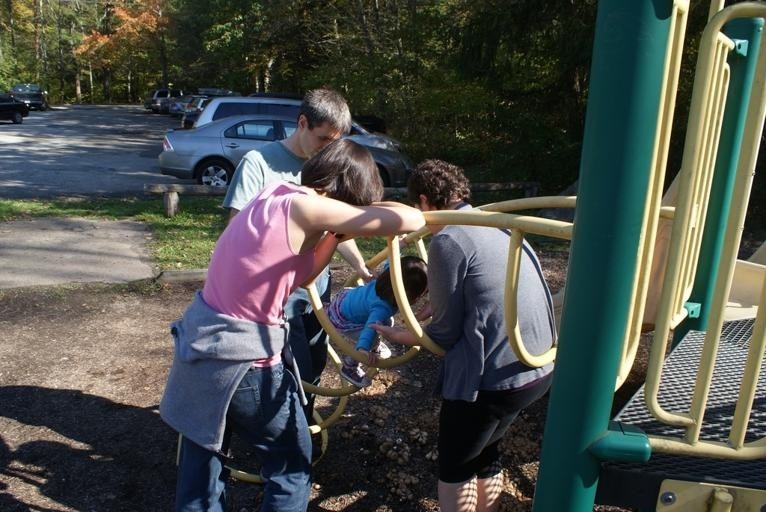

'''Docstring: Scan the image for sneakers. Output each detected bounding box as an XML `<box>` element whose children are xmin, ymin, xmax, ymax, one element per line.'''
<box><xmin>340</xmin><ymin>365</ymin><xmax>369</xmax><ymax>388</ymax></box>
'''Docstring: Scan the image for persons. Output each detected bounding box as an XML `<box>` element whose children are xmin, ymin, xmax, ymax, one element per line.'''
<box><xmin>163</xmin><ymin>88</ymin><xmax>558</xmax><ymax>511</ymax></box>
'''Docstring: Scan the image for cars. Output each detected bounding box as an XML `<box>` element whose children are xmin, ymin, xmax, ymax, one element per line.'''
<box><xmin>144</xmin><ymin>88</ymin><xmax>410</xmax><ymax>186</ymax></box>
<box><xmin>0</xmin><ymin>83</ymin><xmax>48</xmax><ymax>123</ymax></box>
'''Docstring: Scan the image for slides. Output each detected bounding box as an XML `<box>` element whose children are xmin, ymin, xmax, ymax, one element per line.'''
<box><xmin>548</xmin><ymin>171</ymin><xmax>683</xmax><ymax>347</ymax></box>
